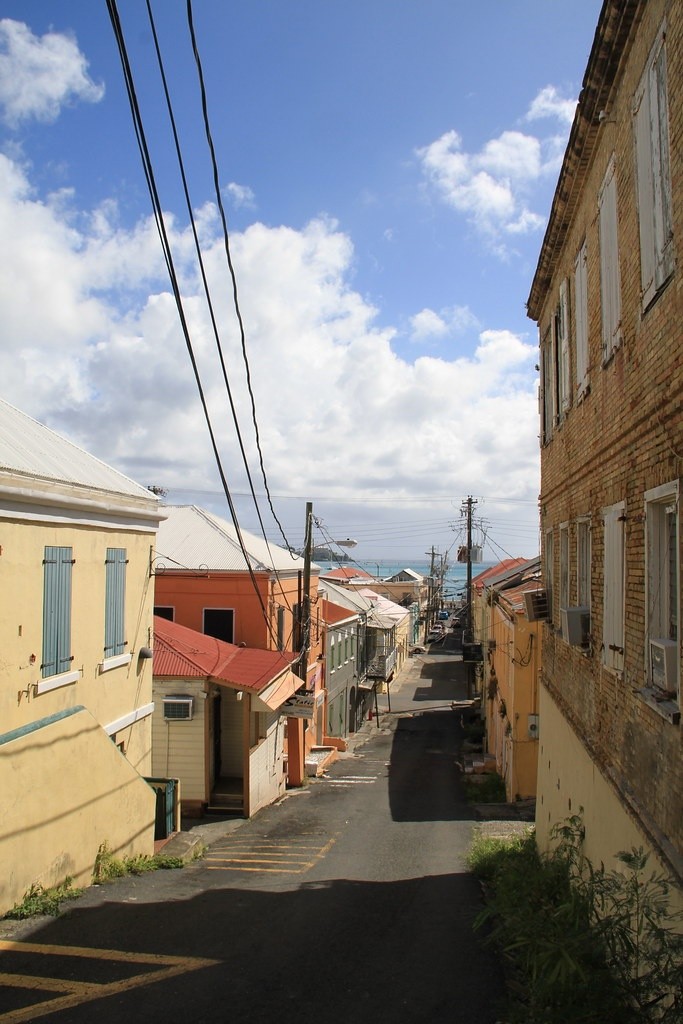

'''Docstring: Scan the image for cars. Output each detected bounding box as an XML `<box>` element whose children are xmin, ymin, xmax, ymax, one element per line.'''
<box><xmin>409</xmin><ymin>644</ymin><xmax>427</xmax><ymax>655</ymax></box>
<box><xmin>429</xmin><ymin>624</ymin><xmax>444</xmax><ymax>642</ymax></box>
<box><xmin>439</xmin><ymin>612</ymin><xmax>449</xmax><ymax>620</ymax></box>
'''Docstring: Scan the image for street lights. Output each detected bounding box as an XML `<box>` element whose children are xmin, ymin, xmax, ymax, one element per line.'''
<box><xmin>299</xmin><ymin>539</ymin><xmax>360</xmax><ymax>769</ymax></box>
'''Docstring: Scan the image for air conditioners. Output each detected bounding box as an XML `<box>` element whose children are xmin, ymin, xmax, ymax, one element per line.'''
<box><xmin>527</xmin><ymin>715</ymin><xmax>539</xmax><ymax>738</ymax></box>
<box><xmin>521</xmin><ymin>588</ymin><xmax>550</xmax><ymax>622</ymax></box>
<box><xmin>559</xmin><ymin>607</ymin><xmax>590</xmax><ymax>646</ymax></box>
<box><xmin>649</xmin><ymin>639</ymin><xmax>677</xmax><ymax>692</ymax></box>
<box><xmin>162</xmin><ymin>697</ymin><xmax>192</xmax><ymax>720</ymax></box>
